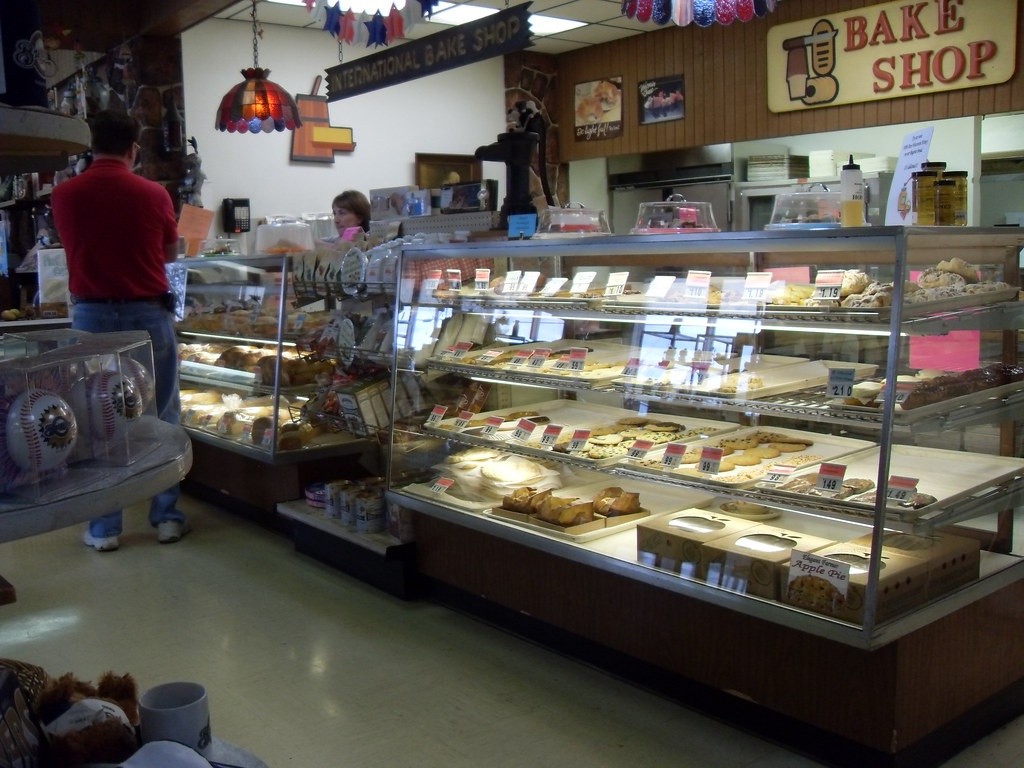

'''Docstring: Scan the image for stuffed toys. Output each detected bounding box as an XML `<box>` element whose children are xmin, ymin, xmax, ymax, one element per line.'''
<box><xmin>38</xmin><ymin>673</ymin><xmax>139</xmax><ymax>758</ymax></box>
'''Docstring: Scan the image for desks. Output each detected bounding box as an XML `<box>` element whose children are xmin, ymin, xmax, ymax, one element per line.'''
<box><xmin>0</xmin><ymin>416</ymin><xmax>194</xmax><ymax>540</ymax></box>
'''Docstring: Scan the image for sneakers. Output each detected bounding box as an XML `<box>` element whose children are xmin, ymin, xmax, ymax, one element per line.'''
<box><xmin>157</xmin><ymin>516</ymin><xmax>190</xmax><ymax>542</ymax></box>
<box><xmin>81</xmin><ymin>530</ymin><xmax>120</xmax><ymax>549</ymax></box>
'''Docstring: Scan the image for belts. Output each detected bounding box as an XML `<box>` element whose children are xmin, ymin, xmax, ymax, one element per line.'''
<box><xmin>73</xmin><ymin>295</ymin><xmax>164</xmax><ymax>305</ymax></box>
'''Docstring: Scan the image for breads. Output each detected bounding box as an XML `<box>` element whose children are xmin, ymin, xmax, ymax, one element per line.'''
<box><xmin>172</xmin><ymin>305</ymin><xmax>335</xmax><ymax>451</ymax></box>
<box><xmin>705</xmin><ymin>253</ymin><xmax>1011</xmax><ymax>311</ymax></box>
<box><xmin>843</xmin><ymin>361</ymin><xmax>1024</xmax><ymax>410</ymax></box>
<box><xmin>488</xmin><ymin>277</ymin><xmax>635</xmax><ymax>297</ymax></box>
<box><xmin>503</xmin><ymin>486</ymin><xmax>641</xmax><ymax>528</ymax></box>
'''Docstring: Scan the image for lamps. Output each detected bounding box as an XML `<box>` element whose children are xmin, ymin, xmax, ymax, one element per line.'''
<box><xmin>215</xmin><ymin>0</ymin><xmax>302</xmax><ymax>134</ymax></box>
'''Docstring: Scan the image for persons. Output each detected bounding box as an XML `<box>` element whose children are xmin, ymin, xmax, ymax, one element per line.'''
<box><xmin>276</xmin><ymin>190</ymin><xmax>371</xmax><ymax>315</ymax></box>
<box><xmin>49</xmin><ymin>115</ymin><xmax>191</xmax><ymax>550</ymax></box>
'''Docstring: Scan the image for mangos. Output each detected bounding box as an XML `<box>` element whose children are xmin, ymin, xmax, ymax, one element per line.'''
<box><xmin>1</xmin><ymin>309</ymin><xmax>21</xmax><ymax>321</ymax></box>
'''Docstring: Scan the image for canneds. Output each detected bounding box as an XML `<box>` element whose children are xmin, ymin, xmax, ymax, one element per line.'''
<box><xmin>910</xmin><ymin>162</ymin><xmax>970</xmax><ymax>228</ymax></box>
<box><xmin>325</xmin><ymin>475</ymin><xmax>395</xmax><ymax>534</ymax></box>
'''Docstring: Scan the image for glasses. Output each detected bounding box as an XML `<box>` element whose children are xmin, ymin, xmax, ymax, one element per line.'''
<box><xmin>135</xmin><ymin>143</ymin><xmax>141</xmax><ymax>151</ymax></box>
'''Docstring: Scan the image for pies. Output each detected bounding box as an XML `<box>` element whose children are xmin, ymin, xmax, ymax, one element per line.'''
<box><xmin>442</xmin><ymin>446</ymin><xmax>543</xmax><ymax>502</ymax></box>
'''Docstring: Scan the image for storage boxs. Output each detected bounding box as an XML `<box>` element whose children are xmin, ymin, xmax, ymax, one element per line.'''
<box><xmin>747</xmin><ymin>154</ymin><xmax>810</xmax><ymax>182</ymax></box>
<box><xmin>491</xmin><ymin>507</ymin><xmax>981</xmax><ymax>630</ymax></box>
<box><xmin>0</xmin><ymin>327</ymin><xmax>159</xmax><ymax>500</ymax></box>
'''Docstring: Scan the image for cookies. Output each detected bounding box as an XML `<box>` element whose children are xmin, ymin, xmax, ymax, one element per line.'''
<box><xmin>774</xmin><ymin>473</ymin><xmax>939</xmax><ymax>514</ymax></box>
<box><xmin>525</xmin><ymin>416</ymin><xmax>822</xmax><ymax>484</ymax></box>
<box><xmin>453</xmin><ymin>346</ymin><xmax>764</xmax><ymax>394</ymax></box>
<box><xmin>439</xmin><ymin>409</ymin><xmax>550</xmax><ymax>432</ymax></box>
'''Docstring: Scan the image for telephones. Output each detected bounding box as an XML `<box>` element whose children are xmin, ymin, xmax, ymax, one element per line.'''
<box><xmin>223</xmin><ymin>198</ymin><xmax>251</xmax><ymax>234</ymax></box>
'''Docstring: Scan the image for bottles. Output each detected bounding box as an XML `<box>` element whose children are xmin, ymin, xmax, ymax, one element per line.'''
<box><xmin>911</xmin><ymin>162</ymin><xmax>968</xmax><ymax>226</ymax></box>
<box><xmin>840</xmin><ymin>155</ymin><xmax>865</xmax><ymax>227</ymax></box>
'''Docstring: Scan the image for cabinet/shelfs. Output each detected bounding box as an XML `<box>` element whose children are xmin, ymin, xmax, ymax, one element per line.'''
<box><xmin>168</xmin><ymin>253</ymin><xmax>396</xmax><ymax>529</ymax></box>
<box><xmin>383</xmin><ymin>224</ymin><xmax>1024</xmax><ymax>656</ymax></box>
